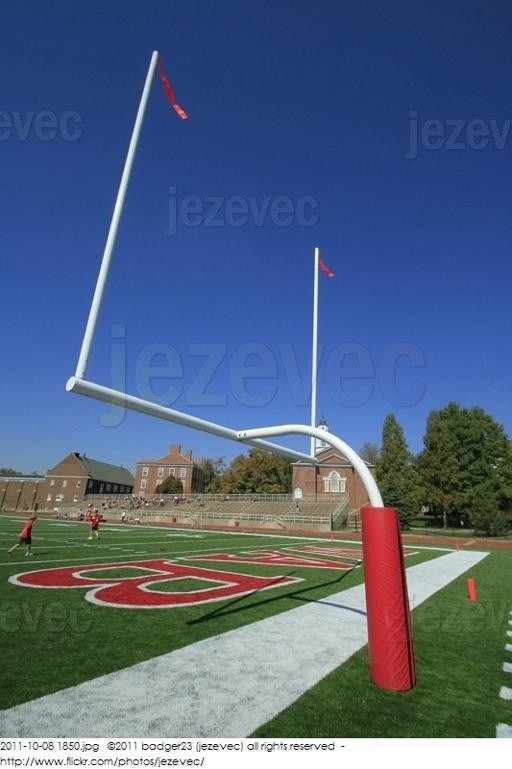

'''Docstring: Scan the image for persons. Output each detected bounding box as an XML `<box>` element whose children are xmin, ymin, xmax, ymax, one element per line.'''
<box><xmin>6</xmin><ymin>514</ymin><xmax>37</xmax><ymax>557</ymax></box>
<box><xmin>78</xmin><ymin>508</ymin><xmax>104</xmax><ymax>541</ymax></box>
<box><xmin>121</xmin><ymin>511</ymin><xmax>126</xmax><ymax>525</ymax></box>
